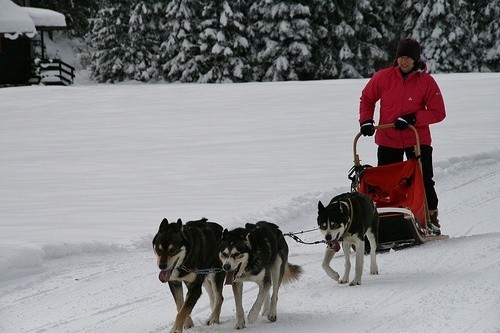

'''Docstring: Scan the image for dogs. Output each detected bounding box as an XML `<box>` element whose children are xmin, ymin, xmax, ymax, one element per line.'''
<box><xmin>217</xmin><ymin>221</ymin><xmax>305</xmax><ymax>330</ymax></box>
<box><xmin>317</xmin><ymin>192</ymin><xmax>380</xmax><ymax>286</ymax></box>
<box><xmin>152</xmin><ymin>217</ymin><xmax>228</xmax><ymax>333</ymax></box>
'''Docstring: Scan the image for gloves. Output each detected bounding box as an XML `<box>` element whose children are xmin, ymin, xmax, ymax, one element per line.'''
<box><xmin>360</xmin><ymin>119</ymin><xmax>375</xmax><ymax>136</ymax></box>
<box><xmin>395</xmin><ymin>114</ymin><xmax>416</xmax><ymax>131</ymax></box>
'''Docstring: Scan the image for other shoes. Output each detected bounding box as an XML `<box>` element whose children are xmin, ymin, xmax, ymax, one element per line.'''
<box><xmin>430</xmin><ymin>210</ymin><xmax>440</xmax><ymax>230</ymax></box>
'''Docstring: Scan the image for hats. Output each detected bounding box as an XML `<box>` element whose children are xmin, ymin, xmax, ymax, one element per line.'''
<box><xmin>396</xmin><ymin>39</ymin><xmax>420</xmax><ymax>60</ymax></box>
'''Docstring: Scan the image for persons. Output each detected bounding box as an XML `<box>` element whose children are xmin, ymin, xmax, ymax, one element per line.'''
<box><xmin>358</xmin><ymin>38</ymin><xmax>447</xmax><ymax>237</ymax></box>
